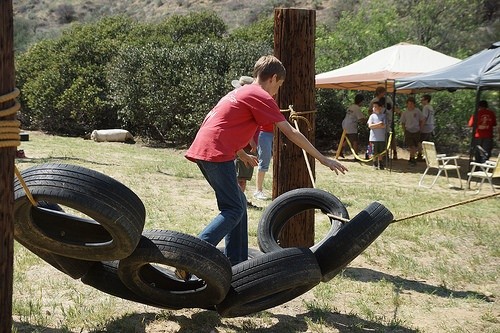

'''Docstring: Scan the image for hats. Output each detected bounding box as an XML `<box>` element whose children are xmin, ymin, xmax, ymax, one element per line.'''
<box><xmin>231</xmin><ymin>76</ymin><xmax>254</xmax><ymax>88</ymax></box>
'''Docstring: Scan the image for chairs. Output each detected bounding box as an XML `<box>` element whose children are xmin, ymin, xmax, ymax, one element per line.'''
<box><xmin>419</xmin><ymin>141</ymin><xmax>462</xmax><ymax>190</ymax></box>
<box><xmin>467</xmin><ymin>153</ymin><xmax>500</xmax><ymax>192</ymax></box>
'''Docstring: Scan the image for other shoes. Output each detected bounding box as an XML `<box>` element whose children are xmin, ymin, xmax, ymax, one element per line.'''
<box><xmin>174</xmin><ymin>268</ymin><xmax>193</xmax><ymax>282</ymax></box>
<box><xmin>340</xmin><ymin>151</ymin><xmax>425</xmax><ymax>170</ymax></box>
<box><xmin>253</xmin><ymin>188</ymin><xmax>269</xmax><ymax>200</ymax></box>
<box><xmin>247</xmin><ymin>201</ymin><xmax>262</xmax><ymax>210</ymax></box>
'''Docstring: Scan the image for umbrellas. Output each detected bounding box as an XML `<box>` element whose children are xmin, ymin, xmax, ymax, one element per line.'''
<box><xmin>314</xmin><ymin>42</ymin><xmax>463</xmax><ymax>93</ymax></box>
<box><xmin>394</xmin><ymin>41</ymin><xmax>500</xmax><ymax>91</ymax></box>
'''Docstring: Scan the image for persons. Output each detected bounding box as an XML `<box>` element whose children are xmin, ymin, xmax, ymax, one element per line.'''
<box><xmin>231</xmin><ymin>75</ymin><xmax>262</xmax><ymax>210</ymax></box>
<box><xmin>184</xmin><ymin>54</ymin><xmax>349</xmax><ymax>269</ymax></box>
<box><xmin>254</xmin><ymin>124</ymin><xmax>274</xmax><ymax>201</ymax></box>
<box><xmin>369</xmin><ymin>87</ymin><xmax>400</xmax><ymax>165</ymax></box>
<box><xmin>400</xmin><ymin>97</ymin><xmax>424</xmax><ymax>166</ymax></box>
<box><xmin>342</xmin><ymin>94</ymin><xmax>367</xmax><ymax>161</ymax></box>
<box><xmin>419</xmin><ymin>95</ymin><xmax>435</xmax><ymax>162</ymax></box>
<box><xmin>468</xmin><ymin>100</ymin><xmax>497</xmax><ymax>177</ymax></box>
<box><xmin>367</xmin><ymin>102</ymin><xmax>389</xmax><ymax>170</ymax></box>
